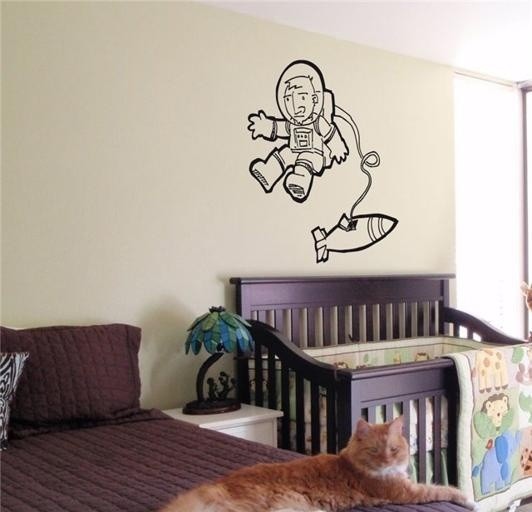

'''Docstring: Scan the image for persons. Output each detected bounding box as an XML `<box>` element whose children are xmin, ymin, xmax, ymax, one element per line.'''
<box><xmin>242</xmin><ymin>58</ymin><xmax>353</xmax><ymax>206</ymax></box>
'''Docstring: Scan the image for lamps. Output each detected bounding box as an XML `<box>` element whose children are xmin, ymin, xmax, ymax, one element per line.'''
<box><xmin>182</xmin><ymin>305</ymin><xmax>256</xmax><ymax>415</ymax></box>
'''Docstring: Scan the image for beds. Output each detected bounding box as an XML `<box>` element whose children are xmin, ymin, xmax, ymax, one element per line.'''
<box><xmin>0</xmin><ymin>412</ymin><xmax>478</xmax><ymax>512</ymax></box>
<box><xmin>228</xmin><ymin>272</ymin><xmax>532</xmax><ymax>486</ymax></box>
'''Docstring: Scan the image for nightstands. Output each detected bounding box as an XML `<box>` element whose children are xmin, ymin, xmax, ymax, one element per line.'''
<box><xmin>160</xmin><ymin>403</ymin><xmax>284</xmax><ymax>450</ymax></box>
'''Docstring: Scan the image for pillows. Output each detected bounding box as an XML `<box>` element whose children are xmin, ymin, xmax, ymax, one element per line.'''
<box><xmin>0</xmin><ymin>323</ymin><xmax>146</xmax><ymax>440</ymax></box>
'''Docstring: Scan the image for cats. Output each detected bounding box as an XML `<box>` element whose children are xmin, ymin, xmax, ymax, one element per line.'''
<box><xmin>154</xmin><ymin>413</ymin><xmax>486</xmax><ymax>512</ymax></box>
